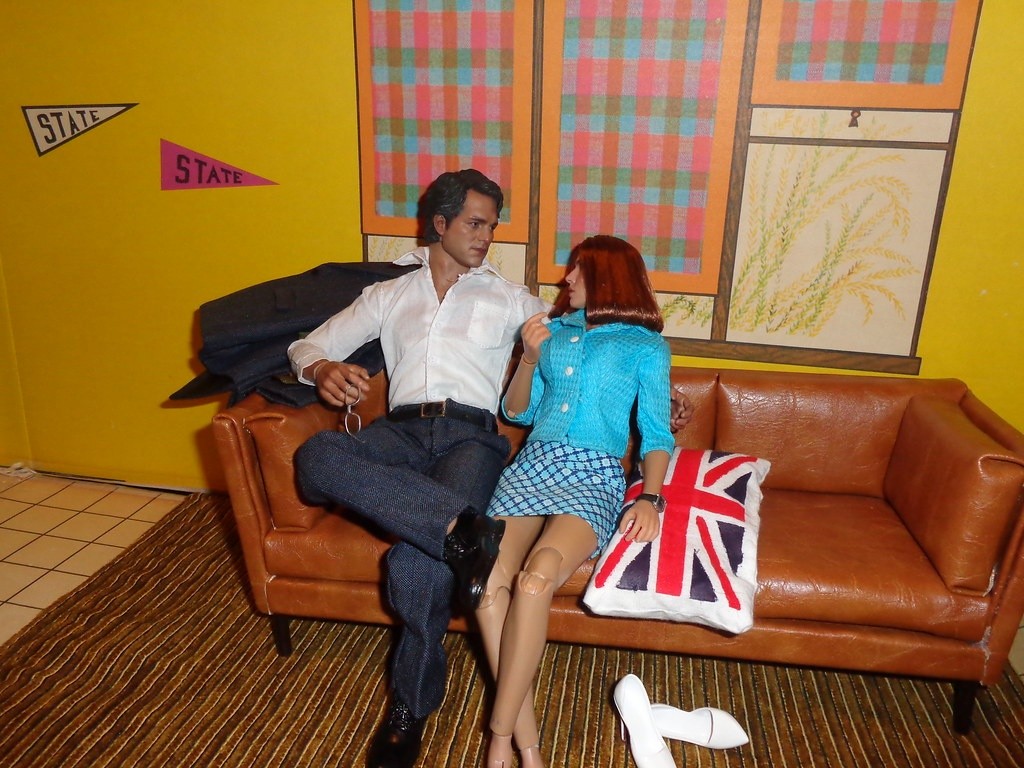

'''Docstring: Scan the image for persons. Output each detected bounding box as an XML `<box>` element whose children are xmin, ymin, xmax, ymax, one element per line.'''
<box><xmin>473</xmin><ymin>234</ymin><xmax>676</xmax><ymax>768</ymax></box>
<box><xmin>286</xmin><ymin>168</ymin><xmax>693</xmax><ymax>768</ymax></box>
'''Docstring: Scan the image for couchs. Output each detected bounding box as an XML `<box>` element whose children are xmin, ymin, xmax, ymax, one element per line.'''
<box><xmin>210</xmin><ymin>364</ymin><xmax>1024</xmax><ymax>735</ymax></box>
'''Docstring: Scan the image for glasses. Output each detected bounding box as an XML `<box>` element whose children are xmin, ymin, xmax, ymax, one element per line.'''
<box><xmin>345</xmin><ymin>385</ymin><xmax>365</xmax><ymax>443</ymax></box>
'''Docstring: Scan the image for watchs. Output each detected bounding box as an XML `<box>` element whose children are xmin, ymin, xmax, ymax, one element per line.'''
<box><xmin>636</xmin><ymin>494</ymin><xmax>666</xmax><ymax>513</ymax></box>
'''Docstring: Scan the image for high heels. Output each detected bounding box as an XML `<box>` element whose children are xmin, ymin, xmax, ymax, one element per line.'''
<box><xmin>651</xmin><ymin>703</ymin><xmax>749</xmax><ymax>750</ymax></box>
<box><xmin>613</xmin><ymin>674</ymin><xmax>677</xmax><ymax>768</ymax></box>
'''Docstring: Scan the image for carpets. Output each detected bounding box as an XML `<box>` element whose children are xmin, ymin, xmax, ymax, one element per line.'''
<box><xmin>0</xmin><ymin>493</ymin><xmax>1024</xmax><ymax>768</ymax></box>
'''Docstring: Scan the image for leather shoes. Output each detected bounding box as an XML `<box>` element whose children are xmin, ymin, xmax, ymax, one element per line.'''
<box><xmin>367</xmin><ymin>693</ymin><xmax>428</xmax><ymax>768</ymax></box>
<box><xmin>443</xmin><ymin>505</ymin><xmax>507</xmax><ymax>613</ymax></box>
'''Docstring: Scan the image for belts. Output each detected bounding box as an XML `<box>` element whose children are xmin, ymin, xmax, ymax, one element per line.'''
<box><xmin>387</xmin><ymin>401</ymin><xmax>499</xmax><ymax>436</ymax></box>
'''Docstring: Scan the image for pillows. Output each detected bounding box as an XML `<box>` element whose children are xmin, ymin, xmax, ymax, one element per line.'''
<box><xmin>581</xmin><ymin>448</ymin><xmax>771</xmax><ymax>636</ymax></box>
